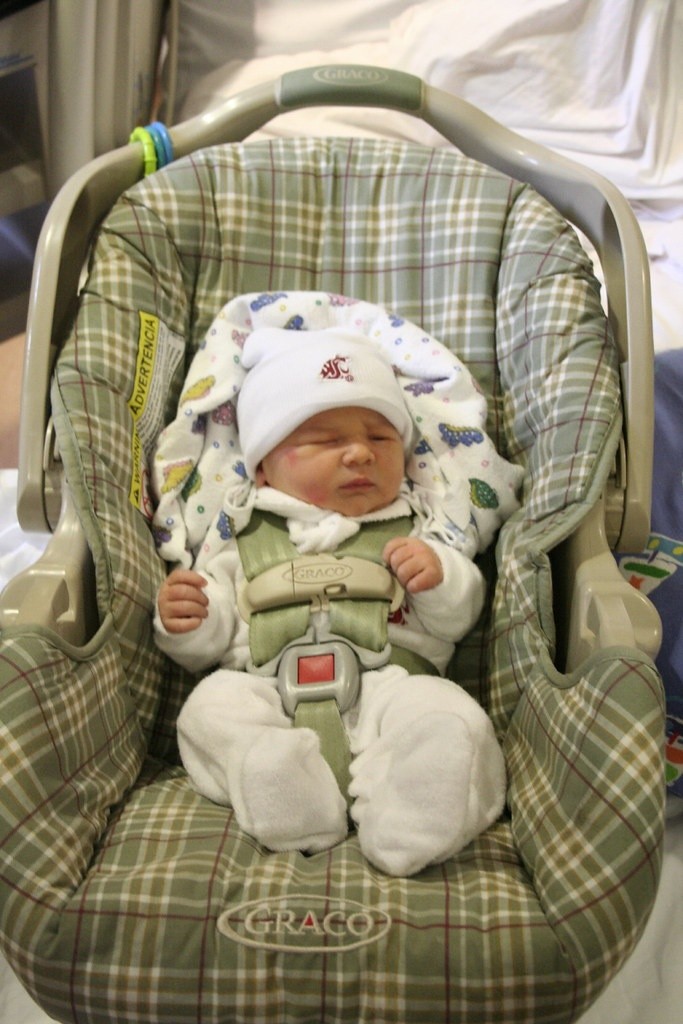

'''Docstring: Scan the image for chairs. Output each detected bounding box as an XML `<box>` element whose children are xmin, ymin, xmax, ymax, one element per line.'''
<box><xmin>1</xmin><ymin>64</ymin><xmax>665</xmax><ymax>1024</ymax></box>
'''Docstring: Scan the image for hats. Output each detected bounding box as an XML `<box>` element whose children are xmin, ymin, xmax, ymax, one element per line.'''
<box><xmin>237</xmin><ymin>327</ymin><xmax>413</xmax><ymax>481</ymax></box>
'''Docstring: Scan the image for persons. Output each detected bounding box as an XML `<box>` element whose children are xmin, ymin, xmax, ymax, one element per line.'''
<box><xmin>153</xmin><ymin>327</ymin><xmax>507</xmax><ymax>875</ymax></box>
<box><xmin>0</xmin><ymin>222</ymin><xmax>33</xmax><ymax>470</ymax></box>
